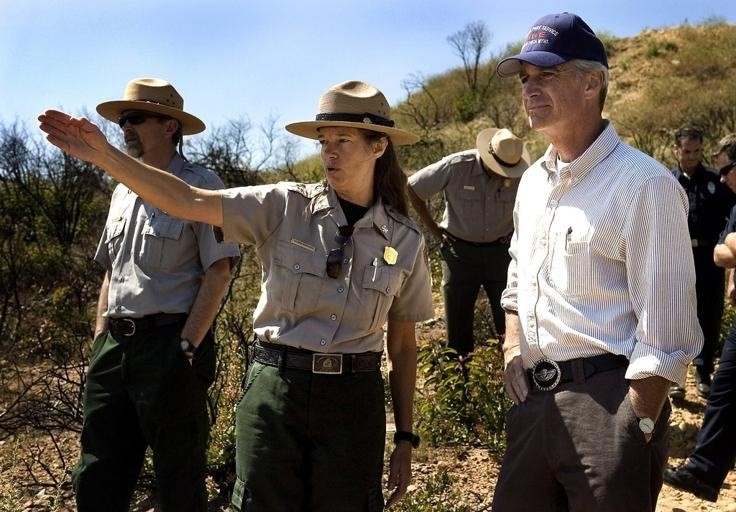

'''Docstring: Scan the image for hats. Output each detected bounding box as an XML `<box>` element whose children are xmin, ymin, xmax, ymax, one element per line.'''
<box><xmin>96</xmin><ymin>77</ymin><xmax>205</xmax><ymax>134</ymax></box>
<box><xmin>476</xmin><ymin>128</ymin><xmax>528</xmax><ymax>178</ymax></box>
<box><xmin>285</xmin><ymin>79</ymin><xmax>421</xmax><ymax>145</ymax></box>
<box><xmin>497</xmin><ymin>12</ymin><xmax>608</xmax><ymax>78</ymax></box>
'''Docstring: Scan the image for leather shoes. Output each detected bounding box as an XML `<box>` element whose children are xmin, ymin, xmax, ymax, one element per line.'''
<box><xmin>664</xmin><ymin>468</ymin><xmax>718</xmax><ymax>503</ymax></box>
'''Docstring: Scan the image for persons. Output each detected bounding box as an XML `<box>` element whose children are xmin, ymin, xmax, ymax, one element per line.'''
<box><xmin>406</xmin><ymin>128</ymin><xmax>528</xmax><ymax>386</ymax></box>
<box><xmin>491</xmin><ymin>12</ymin><xmax>704</xmax><ymax>508</ymax></box>
<box><xmin>37</xmin><ymin>80</ymin><xmax>436</xmax><ymax>510</ymax></box>
<box><xmin>669</xmin><ymin>128</ymin><xmax>736</xmax><ymax>401</ymax></box>
<box><xmin>662</xmin><ymin>132</ymin><xmax>736</xmax><ymax>503</ymax></box>
<box><xmin>72</xmin><ymin>81</ymin><xmax>241</xmax><ymax>511</ymax></box>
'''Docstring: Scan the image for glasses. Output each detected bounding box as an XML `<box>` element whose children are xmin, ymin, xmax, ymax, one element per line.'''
<box><xmin>119</xmin><ymin>113</ymin><xmax>161</xmax><ymax>128</ymax></box>
<box><xmin>327</xmin><ymin>225</ymin><xmax>354</xmax><ymax>278</ymax></box>
<box><xmin>719</xmin><ymin>162</ymin><xmax>736</xmax><ymax>175</ymax></box>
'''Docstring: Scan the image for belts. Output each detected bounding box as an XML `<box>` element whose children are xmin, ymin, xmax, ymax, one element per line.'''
<box><xmin>254</xmin><ymin>343</ymin><xmax>381</xmax><ymax>374</ymax></box>
<box><xmin>109</xmin><ymin>316</ymin><xmax>178</xmax><ymax>336</ymax></box>
<box><xmin>527</xmin><ymin>353</ymin><xmax>624</xmax><ymax>392</ymax></box>
<box><xmin>692</xmin><ymin>239</ymin><xmax>718</xmax><ymax>247</ymax></box>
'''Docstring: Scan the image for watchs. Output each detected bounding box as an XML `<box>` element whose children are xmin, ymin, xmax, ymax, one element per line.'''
<box><xmin>635</xmin><ymin>417</ymin><xmax>657</xmax><ymax>435</ymax></box>
<box><xmin>179</xmin><ymin>337</ymin><xmax>197</xmax><ymax>353</ymax></box>
<box><xmin>394</xmin><ymin>431</ymin><xmax>421</xmax><ymax>449</ymax></box>
